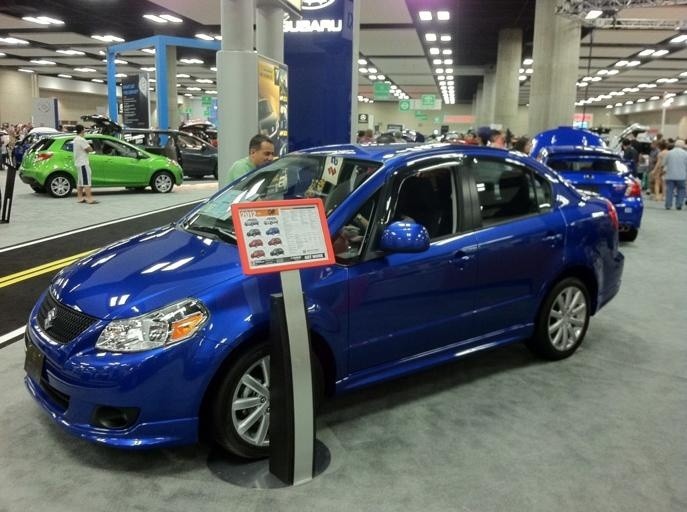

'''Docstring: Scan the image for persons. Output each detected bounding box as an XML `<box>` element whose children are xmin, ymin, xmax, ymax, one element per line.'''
<box><xmin>353</xmin><ymin>182</ymin><xmax>415</xmax><ymax>231</ymax></box>
<box><xmin>225</xmin><ymin>135</ymin><xmax>274</xmax><ymax>186</ymax></box>
<box><xmin>357</xmin><ymin>128</ymin><xmax>532</xmax><ymax>155</ymax></box>
<box><xmin>72</xmin><ymin>124</ymin><xmax>99</xmax><ymax>205</ymax></box>
<box><xmin>621</xmin><ymin>131</ymin><xmax>687</xmax><ymax>210</ymax></box>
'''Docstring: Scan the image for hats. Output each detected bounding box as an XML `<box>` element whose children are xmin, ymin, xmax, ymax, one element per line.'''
<box><xmin>674</xmin><ymin>140</ymin><xmax>686</xmax><ymax>148</ymax></box>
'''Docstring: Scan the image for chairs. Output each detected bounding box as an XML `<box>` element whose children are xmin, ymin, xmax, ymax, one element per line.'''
<box><xmin>487</xmin><ymin>170</ymin><xmax>538</xmax><ymax>219</ymax></box>
<box><xmin>397</xmin><ymin>175</ymin><xmax>442</xmax><ymax>238</ymax></box>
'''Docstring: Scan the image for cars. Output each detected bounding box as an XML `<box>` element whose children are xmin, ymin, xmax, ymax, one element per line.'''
<box><xmin>371</xmin><ymin>127</ymin><xmax>473</xmax><ymax>144</ymax></box>
<box><xmin>250</xmin><ymin>250</ymin><xmax>265</xmax><ymax>258</ymax></box>
<box><xmin>22</xmin><ymin>142</ymin><xmax>626</xmax><ymax>460</ymax></box>
<box><xmin>18</xmin><ymin>136</ymin><xmax>184</xmax><ymax>198</ymax></box>
<box><xmin>263</xmin><ymin>216</ymin><xmax>279</xmax><ymax>225</ymax></box>
<box><xmin>246</xmin><ymin>229</ymin><xmax>261</xmax><ymax>237</ymax></box>
<box><xmin>523</xmin><ymin>125</ymin><xmax>644</xmax><ymax>242</ymax></box>
<box><xmin>608</xmin><ymin>121</ymin><xmax>651</xmax><ymax>176</ymax></box>
<box><xmin>176</xmin><ymin>118</ymin><xmax>217</xmax><ymax>147</ymax></box>
<box><xmin>267</xmin><ymin>238</ymin><xmax>282</xmax><ymax>246</ymax></box>
<box><xmin>243</xmin><ymin>218</ymin><xmax>259</xmax><ymax>226</ymax></box>
<box><xmin>269</xmin><ymin>248</ymin><xmax>284</xmax><ymax>256</ymax></box>
<box><xmin>258</xmin><ymin>96</ymin><xmax>281</xmax><ymax>143</ymax></box>
<box><xmin>80</xmin><ymin>114</ymin><xmax>218</xmax><ymax>182</ymax></box>
<box><xmin>11</xmin><ymin>127</ymin><xmax>75</xmax><ymax>170</ymax></box>
<box><xmin>248</xmin><ymin>239</ymin><xmax>264</xmax><ymax>248</ymax></box>
<box><xmin>265</xmin><ymin>227</ymin><xmax>280</xmax><ymax>235</ymax></box>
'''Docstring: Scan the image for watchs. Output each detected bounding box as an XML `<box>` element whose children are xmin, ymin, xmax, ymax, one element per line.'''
<box><xmin>354</xmin><ymin>214</ymin><xmax>363</xmax><ymax>225</ymax></box>
